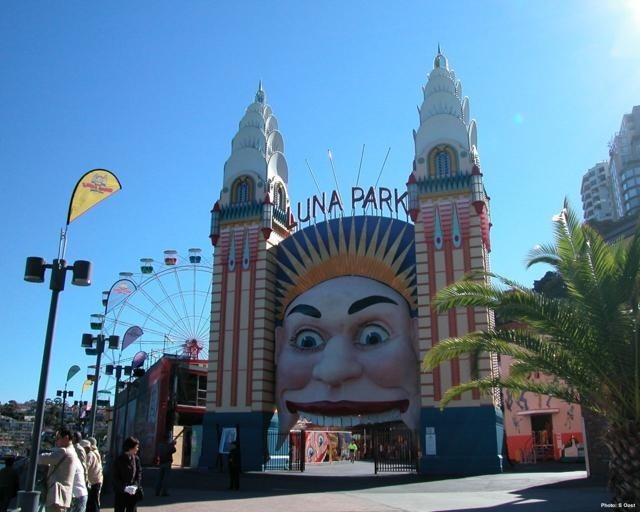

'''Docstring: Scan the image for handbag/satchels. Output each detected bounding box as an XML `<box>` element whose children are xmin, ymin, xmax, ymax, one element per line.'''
<box><xmin>36</xmin><ymin>477</ymin><xmax>48</xmax><ymax>505</ymax></box>
<box><xmin>133</xmin><ymin>487</ymin><xmax>145</xmax><ymax>502</ymax></box>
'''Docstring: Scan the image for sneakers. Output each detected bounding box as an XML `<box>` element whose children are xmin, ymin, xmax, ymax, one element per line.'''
<box><xmin>154</xmin><ymin>490</ymin><xmax>170</xmax><ymax>496</ymax></box>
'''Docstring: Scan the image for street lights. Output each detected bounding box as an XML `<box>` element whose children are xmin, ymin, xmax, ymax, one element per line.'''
<box><xmin>23</xmin><ymin>256</ymin><xmax>148</xmax><ymax>512</ymax></box>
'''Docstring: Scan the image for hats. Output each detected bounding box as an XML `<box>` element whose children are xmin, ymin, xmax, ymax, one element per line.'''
<box><xmin>80</xmin><ymin>437</ymin><xmax>98</xmax><ymax>449</ymax></box>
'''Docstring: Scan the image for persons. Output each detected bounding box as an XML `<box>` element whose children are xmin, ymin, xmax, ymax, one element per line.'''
<box><xmin>341</xmin><ymin>436</ymin><xmax>415</xmax><ymax>466</ymax></box>
<box><xmin>0</xmin><ymin>400</ymin><xmax>114</xmax><ymax>512</ymax></box>
<box><xmin>227</xmin><ymin>441</ymin><xmax>241</xmax><ymax>492</ymax></box>
<box><xmin>155</xmin><ymin>434</ymin><xmax>177</xmax><ymax>497</ymax></box>
<box><xmin>112</xmin><ymin>436</ymin><xmax>143</xmax><ymax>512</ymax></box>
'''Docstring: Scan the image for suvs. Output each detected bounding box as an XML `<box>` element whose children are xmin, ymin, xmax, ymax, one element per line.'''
<box><xmin>0</xmin><ymin>444</ymin><xmax>18</xmax><ymax>461</ymax></box>
